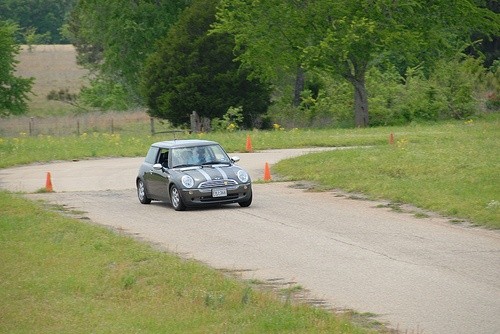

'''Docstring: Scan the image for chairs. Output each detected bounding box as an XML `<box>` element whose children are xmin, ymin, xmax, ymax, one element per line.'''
<box><xmin>162</xmin><ymin>151</ymin><xmax>178</xmax><ymax>168</ymax></box>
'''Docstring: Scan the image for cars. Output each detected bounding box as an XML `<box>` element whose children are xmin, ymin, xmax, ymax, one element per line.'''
<box><xmin>136</xmin><ymin>139</ymin><xmax>253</xmax><ymax>211</ymax></box>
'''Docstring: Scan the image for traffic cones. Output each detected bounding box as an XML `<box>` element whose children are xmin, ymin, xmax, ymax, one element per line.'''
<box><xmin>263</xmin><ymin>163</ymin><xmax>272</xmax><ymax>180</ymax></box>
<box><xmin>389</xmin><ymin>132</ymin><xmax>395</xmax><ymax>145</ymax></box>
<box><xmin>246</xmin><ymin>135</ymin><xmax>252</xmax><ymax>150</ymax></box>
<box><xmin>45</xmin><ymin>172</ymin><xmax>53</xmax><ymax>191</ymax></box>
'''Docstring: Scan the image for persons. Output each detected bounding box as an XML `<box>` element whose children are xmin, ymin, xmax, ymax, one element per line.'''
<box><xmin>189</xmin><ymin>148</ymin><xmax>209</xmax><ymax>165</ymax></box>
